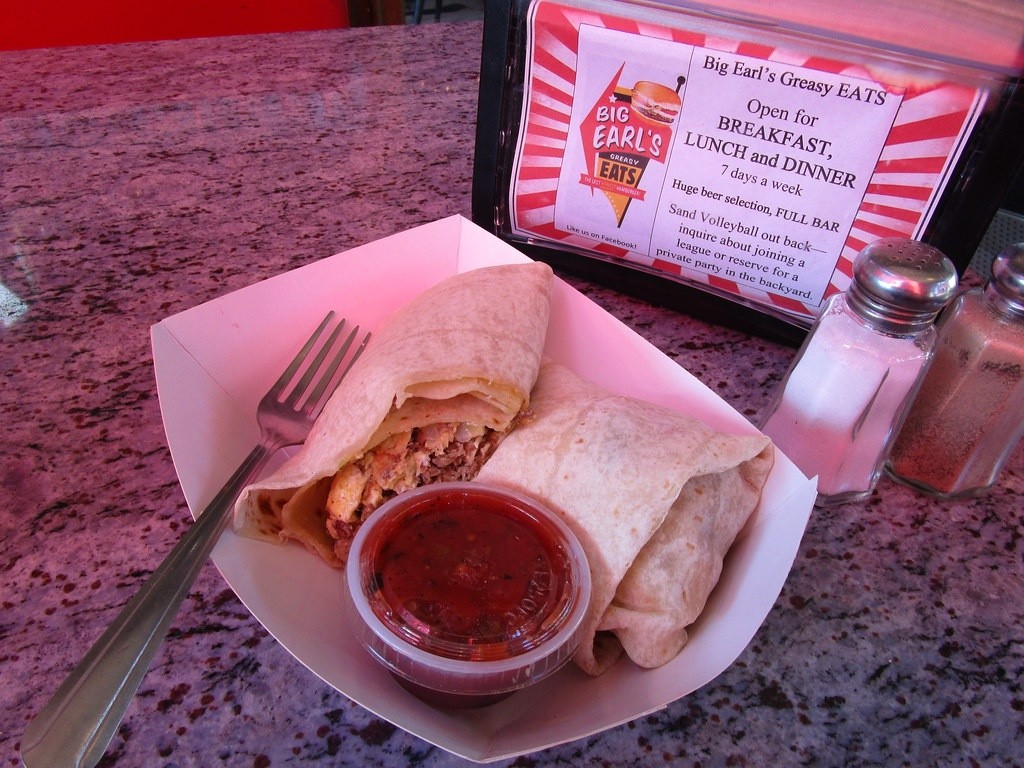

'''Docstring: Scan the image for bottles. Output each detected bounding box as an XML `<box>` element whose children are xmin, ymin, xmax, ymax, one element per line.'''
<box><xmin>762</xmin><ymin>239</ymin><xmax>957</xmax><ymax>505</ymax></box>
<box><xmin>885</xmin><ymin>244</ymin><xmax>1024</xmax><ymax>495</ymax></box>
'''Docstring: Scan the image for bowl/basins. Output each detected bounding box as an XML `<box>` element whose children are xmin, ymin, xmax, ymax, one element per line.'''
<box><xmin>349</xmin><ymin>480</ymin><xmax>593</xmax><ymax>713</ymax></box>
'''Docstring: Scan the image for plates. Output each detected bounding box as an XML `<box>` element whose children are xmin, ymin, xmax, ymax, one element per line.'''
<box><xmin>148</xmin><ymin>217</ymin><xmax>818</xmax><ymax>763</ymax></box>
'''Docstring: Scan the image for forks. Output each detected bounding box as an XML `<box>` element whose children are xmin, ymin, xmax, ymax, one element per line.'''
<box><xmin>10</xmin><ymin>312</ymin><xmax>375</xmax><ymax>768</ymax></box>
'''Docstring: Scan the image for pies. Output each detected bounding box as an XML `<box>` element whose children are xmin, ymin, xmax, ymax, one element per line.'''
<box><xmin>473</xmin><ymin>360</ymin><xmax>773</xmax><ymax>676</ymax></box>
<box><xmin>235</xmin><ymin>261</ymin><xmax>553</xmax><ymax>564</ymax></box>
